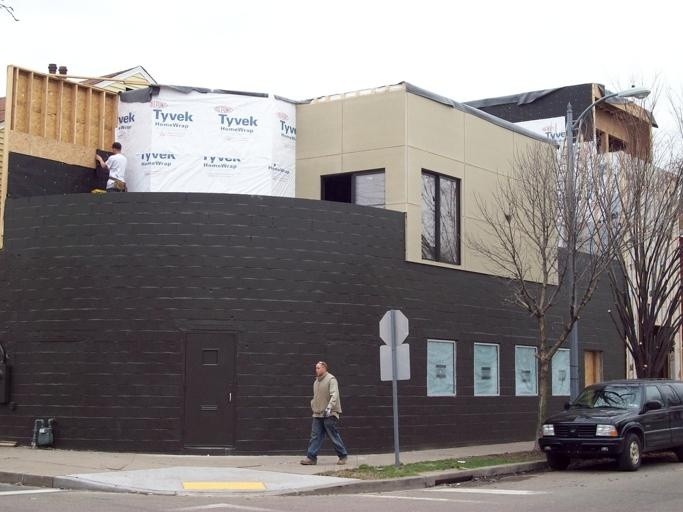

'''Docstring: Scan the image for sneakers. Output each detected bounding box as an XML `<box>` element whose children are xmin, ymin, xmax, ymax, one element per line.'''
<box><xmin>337</xmin><ymin>457</ymin><xmax>347</xmax><ymax>465</ymax></box>
<box><xmin>300</xmin><ymin>458</ymin><xmax>317</xmax><ymax>465</ymax></box>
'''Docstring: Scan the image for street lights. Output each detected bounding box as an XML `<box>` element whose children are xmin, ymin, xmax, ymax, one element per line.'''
<box><xmin>566</xmin><ymin>87</ymin><xmax>651</xmax><ymax>407</ymax></box>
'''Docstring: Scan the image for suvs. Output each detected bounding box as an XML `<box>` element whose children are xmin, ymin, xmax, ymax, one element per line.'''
<box><xmin>536</xmin><ymin>379</ymin><xmax>683</xmax><ymax>469</ymax></box>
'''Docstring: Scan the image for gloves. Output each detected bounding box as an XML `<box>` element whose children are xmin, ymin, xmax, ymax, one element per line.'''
<box><xmin>324</xmin><ymin>409</ymin><xmax>330</xmax><ymax>417</ymax></box>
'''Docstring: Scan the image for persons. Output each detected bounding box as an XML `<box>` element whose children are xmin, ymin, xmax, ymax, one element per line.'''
<box><xmin>95</xmin><ymin>142</ymin><xmax>127</xmax><ymax>193</ymax></box>
<box><xmin>297</xmin><ymin>360</ymin><xmax>349</xmax><ymax>465</ymax></box>
